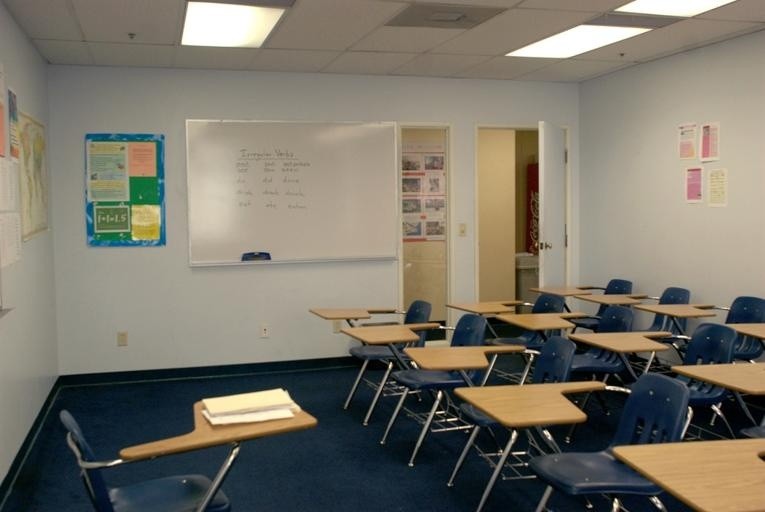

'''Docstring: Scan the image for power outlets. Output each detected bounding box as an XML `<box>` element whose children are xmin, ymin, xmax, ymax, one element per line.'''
<box><xmin>259</xmin><ymin>326</ymin><xmax>272</xmax><ymax>338</ymax></box>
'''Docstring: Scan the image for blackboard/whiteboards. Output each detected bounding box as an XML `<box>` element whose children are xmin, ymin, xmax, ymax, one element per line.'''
<box><xmin>184</xmin><ymin>119</ymin><xmax>398</xmax><ymax>268</ymax></box>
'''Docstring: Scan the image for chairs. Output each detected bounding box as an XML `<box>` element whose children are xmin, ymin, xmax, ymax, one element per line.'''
<box><xmin>488</xmin><ymin>293</ymin><xmax>565</xmax><ymax>355</ymax></box>
<box><xmin>59</xmin><ymin>388</ymin><xmax>230</xmax><ymax>512</ymax></box>
<box><xmin>663</xmin><ymin>323</ymin><xmax>738</xmax><ymax>438</ymax></box>
<box><xmin>343</xmin><ymin>299</ymin><xmax>430</xmax><ymax>426</ymax></box>
<box><xmin>379</xmin><ymin>314</ymin><xmax>486</xmax><ymax>468</ymax></box>
<box><xmin>645</xmin><ymin>287</ymin><xmax>690</xmax><ymax>371</ymax></box>
<box><xmin>528</xmin><ymin>372</ymin><xmax>690</xmax><ymax>512</ymax></box>
<box><xmin>724</xmin><ymin>296</ymin><xmax>765</xmax><ymax>362</ymax></box>
<box><xmin>446</xmin><ymin>337</ymin><xmax>575</xmax><ymax>487</ymax></box>
<box><xmin>567</xmin><ymin>279</ymin><xmax>632</xmax><ymax>332</ymax></box>
<box><xmin>570</xmin><ymin>306</ymin><xmax>634</xmax><ymax>411</ymax></box>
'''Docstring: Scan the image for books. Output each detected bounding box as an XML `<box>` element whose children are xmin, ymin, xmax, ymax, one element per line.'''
<box><xmin>202</xmin><ymin>387</ymin><xmax>294</xmax><ymax>427</ymax></box>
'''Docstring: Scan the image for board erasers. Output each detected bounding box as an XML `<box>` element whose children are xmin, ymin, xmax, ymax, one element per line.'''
<box><xmin>242</xmin><ymin>252</ymin><xmax>271</xmax><ymax>261</ymax></box>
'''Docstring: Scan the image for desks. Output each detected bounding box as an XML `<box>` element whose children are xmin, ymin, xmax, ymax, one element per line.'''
<box><xmin>610</xmin><ymin>438</ymin><xmax>765</xmax><ymax>512</ymax></box>
<box><xmin>634</xmin><ymin>303</ymin><xmax>717</xmax><ymax>318</ymax></box>
<box><xmin>671</xmin><ymin>362</ymin><xmax>765</xmax><ymax>396</ymax></box>
<box><xmin>529</xmin><ymin>286</ymin><xmax>607</xmax><ymax>297</ymax></box>
<box><xmin>453</xmin><ymin>381</ymin><xmax>606</xmax><ymax>429</ymax></box>
<box><xmin>568</xmin><ymin>330</ymin><xmax>672</xmax><ymax>353</ymax></box>
<box><xmin>574</xmin><ymin>294</ymin><xmax>649</xmax><ymax>306</ymax></box>
<box><xmin>726</xmin><ymin>323</ymin><xmax>765</xmax><ymax>339</ymax></box>
<box><xmin>310</xmin><ymin>307</ymin><xmax>396</xmax><ymax>320</ymax></box>
<box><xmin>446</xmin><ymin>300</ymin><xmax>524</xmax><ymax>315</ymax></box>
<box><xmin>403</xmin><ymin>344</ymin><xmax>526</xmax><ymax>370</ymax></box>
<box><xmin>343</xmin><ymin>322</ymin><xmax>440</xmax><ymax>345</ymax></box>
<box><xmin>119</xmin><ymin>391</ymin><xmax>317</xmax><ymax>463</ymax></box>
<box><xmin>496</xmin><ymin>312</ymin><xmax>586</xmax><ymax>332</ymax></box>
